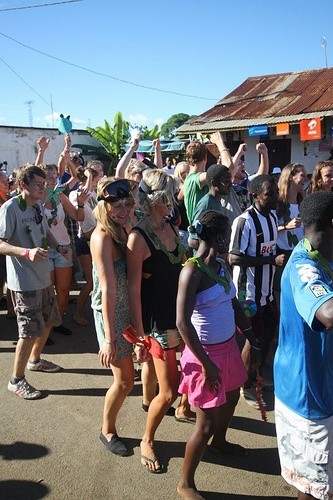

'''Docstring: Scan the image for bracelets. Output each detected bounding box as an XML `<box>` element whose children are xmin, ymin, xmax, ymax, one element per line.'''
<box><xmin>25</xmin><ymin>247</ymin><xmax>29</xmax><ymax>261</ymax></box>
<box><xmin>105</xmin><ymin>338</ymin><xmax>118</xmax><ymax>345</ymax></box>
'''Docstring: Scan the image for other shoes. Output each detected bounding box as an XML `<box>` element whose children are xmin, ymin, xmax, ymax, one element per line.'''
<box><xmin>99</xmin><ymin>431</ymin><xmax>133</xmax><ymax>457</ymax></box>
<box><xmin>54</xmin><ymin>326</ymin><xmax>72</xmax><ymax>335</ymax></box>
<box><xmin>240</xmin><ymin>384</ymin><xmax>267</xmax><ymax>410</ymax></box>
<box><xmin>46</xmin><ymin>338</ymin><xmax>54</xmax><ymax>346</ymax></box>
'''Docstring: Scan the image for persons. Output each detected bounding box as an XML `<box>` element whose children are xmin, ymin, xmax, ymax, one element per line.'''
<box><xmin>0</xmin><ymin>131</ymin><xmax>333</xmax><ymax>411</ymax></box>
<box><xmin>0</xmin><ymin>166</ymin><xmax>72</xmax><ymax>400</ymax></box>
<box><xmin>176</xmin><ymin>210</ymin><xmax>248</xmax><ymax>500</ymax></box>
<box><xmin>89</xmin><ymin>174</ymin><xmax>135</xmax><ymax>457</ymax></box>
<box><xmin>127</xmin><ymin>169</ymin><xmax>197</xmax><ymax>474</ymax></box>
<box><xmin>273</xmin><ymin>191</ymin><xmax>333</xmax><ymax>500</ymax></box>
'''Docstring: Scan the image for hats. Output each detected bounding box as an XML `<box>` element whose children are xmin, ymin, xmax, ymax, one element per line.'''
<box><xmin>96</xmin><ymin>178</ymin><xmax>132</xmax><ymax>203</ymax></box>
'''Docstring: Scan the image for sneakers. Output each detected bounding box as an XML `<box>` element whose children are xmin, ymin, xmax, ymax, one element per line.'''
<box><xmin>27</xmin><ymin>359</ymin><xmax>61</xmax><ymax>373</ymax></box>
<box><xmin>7</xmin><ymin>378</ymin><xmax>42</xmax><ymax>400</ymax></box>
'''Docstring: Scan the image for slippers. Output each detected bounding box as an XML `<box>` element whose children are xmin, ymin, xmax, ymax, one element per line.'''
<box><xmin>140</xmin><ymin>454</ymin><xmax>164</xmax><ymax>474</ymax></box>
<box><xmin>173</xmin><ymin>411</ymin><xmax>197</xmax><ymax>426</ymax></box>
<box><xmin>72</xmin><ymin>315</ymin><xmax>89</xmax><ymax>328</ymax></box>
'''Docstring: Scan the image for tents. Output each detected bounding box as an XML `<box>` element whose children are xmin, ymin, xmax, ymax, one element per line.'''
<box><xmin>124</xmin><ymin>140</ymin><xmax>190</xmax><ymax>152</ymax></box>
